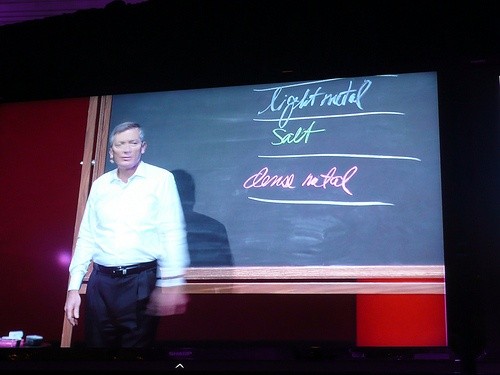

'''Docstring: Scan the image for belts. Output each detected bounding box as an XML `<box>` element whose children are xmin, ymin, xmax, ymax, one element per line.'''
<box><xmin>93</xmin><ymin>260</ymin><xmax>157</xmax><ymax>278</ymax></box>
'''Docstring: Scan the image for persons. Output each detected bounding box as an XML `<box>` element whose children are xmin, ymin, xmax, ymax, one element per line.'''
<box><xmin>64</xmin><ymin>121</ymin><xmax>190</xmax><ymax>375</ymax></box>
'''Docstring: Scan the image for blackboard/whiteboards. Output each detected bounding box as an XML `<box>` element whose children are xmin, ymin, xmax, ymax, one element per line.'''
<box><xmin>81</xmin><ymin>72</ymin><xmax>445</xmax><ymax>280</ymax></box>
